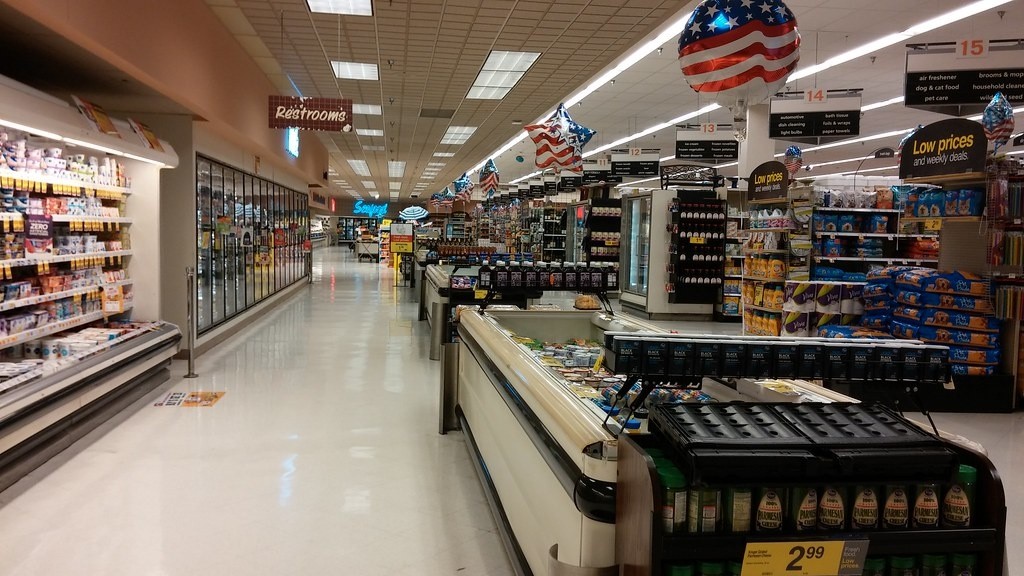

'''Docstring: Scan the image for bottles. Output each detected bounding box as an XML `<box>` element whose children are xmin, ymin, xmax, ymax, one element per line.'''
<box><xmin>749</xmin><ymin>209</ymin><xmax>793</xmax><ymax>227</ymax></box>
<box><xmin>493</xmin><ymin>260</ymin><xmax>508</xmax><ymax>291</ymax></box>
<box><xmin>576</xmin><ymin>262</ymin><xmax>620</xmax><ymax>291</ymax></box>
<box><xmin>549</xmin><ymin>261</ymin><xmax>576</xmax><ymax>290</ymax></box>
<box><xmin>679</xmin><ymin>269</ymin><xmax>721</xmax><ymax>284</ymax></box>
<box><xmin>544</xmin><ymin>237</ymin><xmax>565</xmax><ymax>248</ymax></box>
<box><xmin>745</xmin><ymin>310</ymin><xmax>781</xmax><ymax>336</ymax></box>
<box><xmin>508</xmin><ymin>261</ymin><xmax>548</xmax><ymax>291</ymax></box>
<box><xmin>681</xmin><ymin>204</ymin><xmax>724</xmax><ymax>219</ymax></box>
<box><xmin>744</xmin><ymin>253</ymin><xmax>785</xmax><ymax>278</ymax></box>
<box><xmin>680</xmin><ymin>223</ymin><xmax>724</xmax><ymax>240</ymax></box>
<box><xmin>545</xmin><ymin>209</ymin><xmax>562</xmax><ymax>220</ymax></box>
<box><xmin>742</xmin><ymin>281</ymin><xmax>783</xmax><ymax>311</ymax></box>
<box><xmin>679</xmin><ymin>246</ymin><xmax>723</xmax><ymax>261</ymax></box>
<box><xmin>426</xmin><ymin>237</ymin><xmax>474</xmax><ymax>247</ymax></box>
<box><xmin>479</xmin><ymin>260</ymin><xmax>492</xmax><ymax>290</ymax></box>
<box><xmin>544</xmin><ymin>222</ymin><xmax>561</xmax><ymax>234</ymax></box>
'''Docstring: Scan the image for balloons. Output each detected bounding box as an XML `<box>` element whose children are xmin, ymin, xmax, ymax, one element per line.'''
<box><xmin>430</xmin><ymin>173</ymin><xmax>474</xmax><ymax>209</ymax></box>
<box><xmin>982</xmin><ymin>92</ymin><xmax>1014</xmax><ymax>153</ymax></box>
<box><xmin>524</xmin><ymin>102</ymin><xmax>597</xmax><ymax>176</ymax></box>
<box><xmin>784</xmin><ymin>146</ymin><xmax>803</xmax><ymax>175</ymax></box>
<box><xmin>679</xmin><ymin>0</ymin><xmax>802</xmax><ymax>141</ymax></box>
<box><xmin>897</xmin><ymin>124</ymin><xmax>922</xmax><ymax>168</ymax></box>
<box><xmin>479</xmin><ymin>159</ymin><xmax>499</xmax><ymax>199</ymax></box>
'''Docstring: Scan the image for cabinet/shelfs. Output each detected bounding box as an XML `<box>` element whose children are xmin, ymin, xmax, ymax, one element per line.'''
<box><xmin>427</xmin><ymin>170</ymin><xmax>1024</xmax><ymax>414</ymax></box>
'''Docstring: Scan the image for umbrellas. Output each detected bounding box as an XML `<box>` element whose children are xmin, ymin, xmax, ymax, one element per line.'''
<box><xmin>399</xmin><ymin>205</ymin><xmax>429</xmax><ymax>221</ymax></box>
